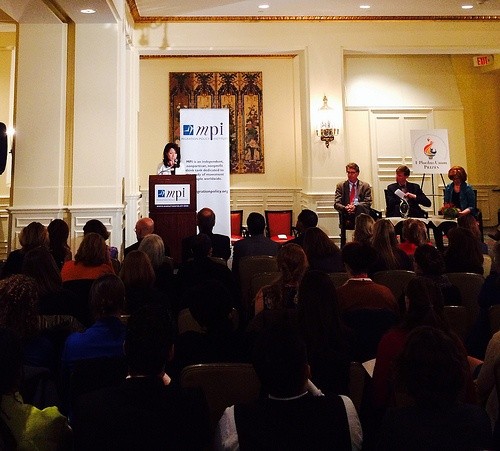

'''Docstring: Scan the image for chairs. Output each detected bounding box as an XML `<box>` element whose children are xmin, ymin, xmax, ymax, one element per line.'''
<box><xmin>385</xmin><ymin>188</ymin><xmax>431</xmax><ymax>241</ymax></box>
<box><xmin>338</xmin><ymin>205</ymin><xmax>382</xmax><ymax>246</ymax></box>
<box><xmin>263</xmin><ymin>210</ymin><xmax>301</xmax><ymax>245</ymax></box>
<box><xmin>173</xmin><ymin>247</ymin><xmax>500</xmax><ymax>437</ymax></box>
<box><xmin>443</xmin><ymin>187</ymin><xmax>484</xmax><ymax>242</ymax></box>
<box><xmin>231</xmin><ymin>209</ymin><xmax>246</xmax><ymax>244</ymax></box>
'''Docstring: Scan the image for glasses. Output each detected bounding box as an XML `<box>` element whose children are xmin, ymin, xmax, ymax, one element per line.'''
<box><xmin>346</xmin><ymin>171</ymin><xmax>356</xmax><ymax>175</ymax></box>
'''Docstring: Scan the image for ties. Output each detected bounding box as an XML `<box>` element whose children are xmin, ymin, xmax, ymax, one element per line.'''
<box><xmin>350</xmin><ymin>184</ymin><xmax>355</xmax><ymax>205</ymax></box>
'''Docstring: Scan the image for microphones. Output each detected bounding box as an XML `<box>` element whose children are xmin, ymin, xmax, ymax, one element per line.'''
<box><xmin>155</xmin><ymin>154</ymin><xmax>175</xmax><ymax>175</ymax></box>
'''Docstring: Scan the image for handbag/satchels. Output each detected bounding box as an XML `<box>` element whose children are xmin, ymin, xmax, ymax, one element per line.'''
<box><xmin>0</xmin><ymin>381</ymin><xmax>72</xmax><ymax>451</ymax></box>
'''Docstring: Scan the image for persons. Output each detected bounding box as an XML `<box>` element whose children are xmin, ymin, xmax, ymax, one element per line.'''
<box><xmin>158</xmin><ymin>143</ymin><xmax>187</xmax><ymax>175</ymax></box>
<box><xmin>379</xmin><ymin>326</ymin><xmax>493</xmax><ymax>451</ymax></box>
<box><xmin>0</xmin><ymin>208</ymin><xmax>500</xmax><ymax>451</ymax></box>
<box><xmin>213</xmin><ymin>323</ymin><xmax>364</xmax><ymax>451</ymax></box>
<box><xmin>488</xmin><ymin>209</ymin><xmax>500</xmax><ymax>241</ymax></box>
<box><xmin>334</xmin><ymin>163</ymin><xmax>372</xmax><ymax>226</ymax></box>
<box><xmin>386</xmin><ymin>165</ymin><xmax>431</xmax><ymax>218</ymax></box>
<box><xmin>444</xmin><ymin>166</ymin><xmax>480</xmax><ymax>232</ymax></box>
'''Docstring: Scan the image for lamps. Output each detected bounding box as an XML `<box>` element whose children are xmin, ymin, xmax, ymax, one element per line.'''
<box><xmin>315</xmin><ymin>94</ymin><xmax>340</xmax><ymax>148</ymax></box>
